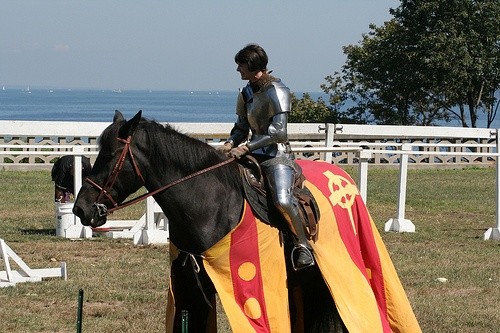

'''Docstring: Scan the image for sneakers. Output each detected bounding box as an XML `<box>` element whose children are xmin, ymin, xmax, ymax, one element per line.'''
<box><xmin>293</xmin><ymin>248</ymin><xmax>313</xmax><ymax>269</ymax></box>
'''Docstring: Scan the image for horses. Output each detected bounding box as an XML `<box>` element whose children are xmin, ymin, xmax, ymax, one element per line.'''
<box><xmin>51</xmin><ymin>155</ymin><xmax>91</xmax><ymax>203</ymax></box>
<box><xmin>73</xmin><ymin>110</ymin><xmax>367</xmax><ymax>332</ymax></box>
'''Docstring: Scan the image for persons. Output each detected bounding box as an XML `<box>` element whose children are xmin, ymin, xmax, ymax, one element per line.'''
<box><xmin>222</xmin><ymin>44</ymin><xmax>313</xmax><ymax>268</ymax></box>
<box><xmin>52</xmin><ymin>155</ymin><xmax>92</xmax><ymax>203</ymax></box>
